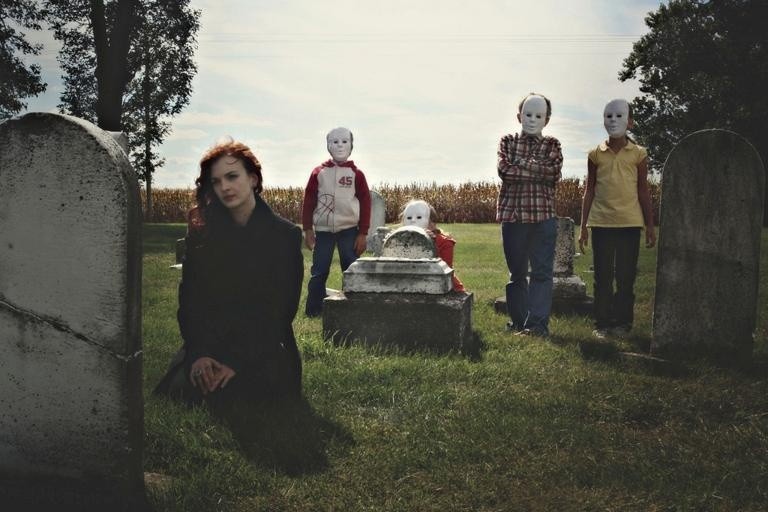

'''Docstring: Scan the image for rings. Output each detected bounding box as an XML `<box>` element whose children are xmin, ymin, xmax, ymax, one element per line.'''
<box><xmin>191</xmin><ymin>368</ymin><xmax>202</xmax><ymax>377</ymax></box>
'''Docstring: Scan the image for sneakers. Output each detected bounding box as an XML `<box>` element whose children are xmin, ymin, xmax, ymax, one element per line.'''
<box><xmin>508</xmin><ymin>321</ymin><xmax>545</xmax><ymax>338</ymax></box>
<box><xmin>594</xmin><ymin>326</ymin><xmax>631</xmax><ymax>340</ymax></box>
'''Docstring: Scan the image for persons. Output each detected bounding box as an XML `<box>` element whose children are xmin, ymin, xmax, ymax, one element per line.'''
<box><xmin>302</xmin><ymin>128</ymin><xmax>371</xmax><ymax>318</ymax></box>
<box><xmin>496</xmin><ymin>93</ymin><xmax>563</xmax><ymax>337</ymax></box>
<box><xmin>152</xmin><ymin>140</ymin><xmax>304</xmax><ymax>443</ymax></box>
<box><xmin>402</xmin><ymin>200</ymin><xmax>466</xmax><ymax>293</ymax></box>
<box><xmin>579</xmin><ymin>99</ymin><xmax>657</xmax><ymax>339</ymax></box>
